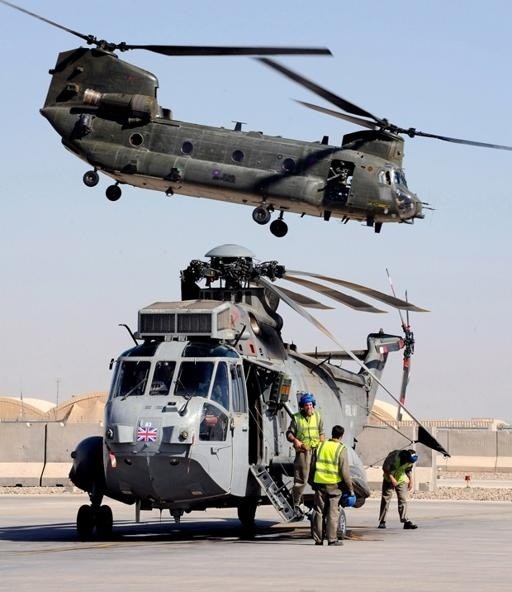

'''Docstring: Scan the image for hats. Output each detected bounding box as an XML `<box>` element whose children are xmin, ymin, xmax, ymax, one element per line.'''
<box><xmin>406</xmin><ymin>449</ymin><xmax>418</xmax><ymax>463</ymax></box>
<box><xmin>340</xmin><ymin>495</ymin><xmax>356</xmax><ymax>508</ymax></box>
<box><xmin>302</xmin><ymin>395</ymin><xmax>314</xmax><ymax>403</ymax></box>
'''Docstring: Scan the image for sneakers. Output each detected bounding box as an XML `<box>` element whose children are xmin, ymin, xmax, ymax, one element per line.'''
<box><xmin>403</xmin><ymin>521</ymin><xmax>417</xmax><ymax>529</ymax></box>
<box><xmin>378</xmin><ymin>521</ymin><xmax>386</xmax><ymax>528</ymax></box>
<box><xmin>328</xmin><ymin>541</ymin><xmax>343</xmax><ymax>546</ymax></box>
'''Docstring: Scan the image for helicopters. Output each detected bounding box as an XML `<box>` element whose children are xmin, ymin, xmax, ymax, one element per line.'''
<box><xmin>1</xmin><ymin>0</ymin><xmax>511</xmax><ymax>239</ymax></box>
<box><xmin>66</xmin><ymin>241</ymin><xmax>455</xmax><ymax>543</ymax></box>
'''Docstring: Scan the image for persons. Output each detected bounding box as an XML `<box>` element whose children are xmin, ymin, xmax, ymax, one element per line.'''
<box><xmin>311</xmin><ymin>425</ymin><xmax>355</xmax><ymax>546</ymax></box>
<box><xmin>378</xmin><ymin>450</ymin><xmax>418</xmax><ymax>529</ymax></box>
<box><xmin>286</xmin><ymin>393</ymin><xmax>324</xmax><ymax>506</ymax></box>
